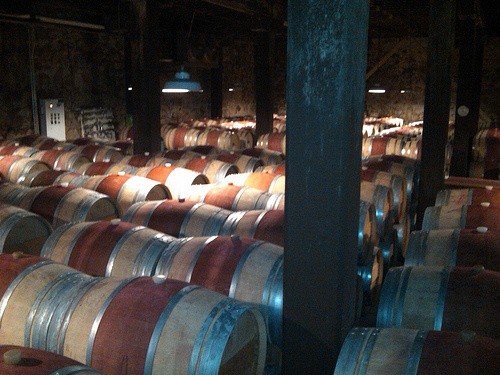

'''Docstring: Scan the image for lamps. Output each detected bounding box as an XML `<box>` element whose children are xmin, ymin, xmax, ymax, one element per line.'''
<box><xmin>369</xmin><ymin>35</ymin><xmax>386</xmax><ymax>93</ymax></box>
<box><xmin>162</xmin><ymin>65</ymin><xmax>203</xmax><ymax>94</ymax></box>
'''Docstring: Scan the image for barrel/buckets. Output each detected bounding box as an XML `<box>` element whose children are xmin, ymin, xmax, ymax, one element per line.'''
<box><xmin>0</xmin><ymin>112</ymin><xmax>500</xmax><ymax>375</ymax></box>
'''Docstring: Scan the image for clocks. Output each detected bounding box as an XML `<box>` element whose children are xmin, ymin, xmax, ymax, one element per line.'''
<box><xmin>457</xmin><ymin>105</ymin><xmax>469</xmax><ymax>117</ymax></box>
